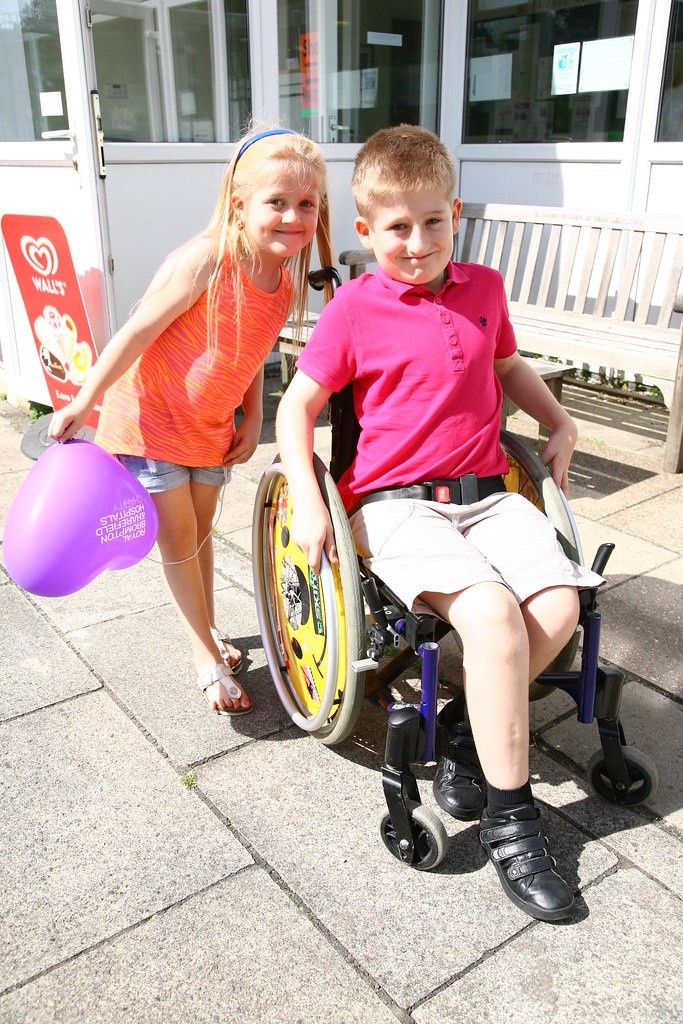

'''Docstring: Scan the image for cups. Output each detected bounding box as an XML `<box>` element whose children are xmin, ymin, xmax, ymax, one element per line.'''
<box><xmin>69</xmin><ymin>349</ymin><xmax>90</xmax><ymax>385</ymax></box>
<box><xmin>41</xmin><ymin>346</ymin><xmax>67</xmax><ymax>382</ymax></box>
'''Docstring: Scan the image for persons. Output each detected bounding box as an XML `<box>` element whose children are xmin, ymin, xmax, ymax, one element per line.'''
<box><xmin>46</xmin><ymin>127</ymin><xmax>326</xmax><ymax>716</ymax></box>
<box><xmin>275</xmin><ymin>124</ymin><xmax>607</xmax><ymax>923</ymax></box>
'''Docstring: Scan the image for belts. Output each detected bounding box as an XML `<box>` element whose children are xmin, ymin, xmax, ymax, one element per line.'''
<box><xmin>356</xmin><ymin>476</ymin><xmax>507</xmax><ymax>505</ymax></box>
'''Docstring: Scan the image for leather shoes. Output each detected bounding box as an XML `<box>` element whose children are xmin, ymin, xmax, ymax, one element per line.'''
<box><xmin>476</xmin><ymin>808</ymin><xmax>574</xmax><ymax>921</ymax></box>
<box><xmin>432</xmin><ymin>711</ymin><xmax>486</xmax><ymax>820</ymax></box>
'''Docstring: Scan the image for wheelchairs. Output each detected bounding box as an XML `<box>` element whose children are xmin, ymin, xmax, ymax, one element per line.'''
<box><xmin>247</xmin><ymin>265</ymin><xmax>660</xmax><ymax>873</ymax></box>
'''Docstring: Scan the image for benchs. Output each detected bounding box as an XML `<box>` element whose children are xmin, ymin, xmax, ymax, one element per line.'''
<box><xmin>269</xmin><ymin>309</ymin><xmax>576</xmax><ymax>451</ymax></box>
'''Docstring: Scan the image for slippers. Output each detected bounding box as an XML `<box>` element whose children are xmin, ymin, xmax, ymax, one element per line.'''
<box><xmin>211</xmin><ymin>626</ymin><xmax>243</xmax><ymax>673</ymax></box>
<box><xmin>194</xmin><ymin>661</ymin><xmax>250</xmax><ymax>716</ymax></box>
